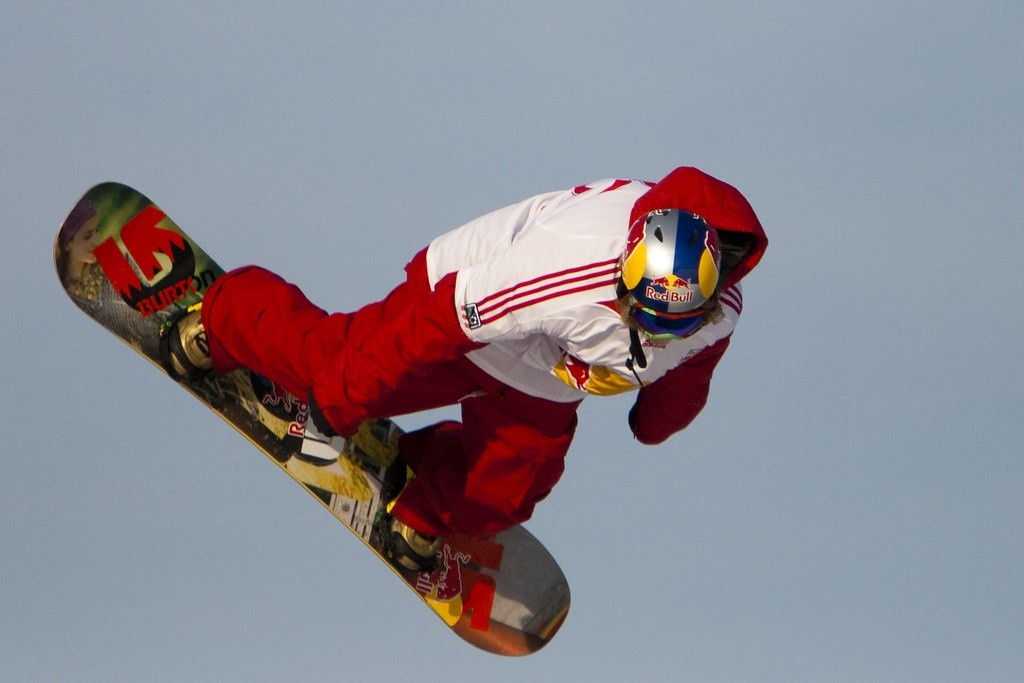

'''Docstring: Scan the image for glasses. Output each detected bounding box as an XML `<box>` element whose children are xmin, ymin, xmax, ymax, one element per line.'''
<box><xmin>629</xmin><ymin>305</ymin><xmax>710</xmax><ymax>340</ymax></box>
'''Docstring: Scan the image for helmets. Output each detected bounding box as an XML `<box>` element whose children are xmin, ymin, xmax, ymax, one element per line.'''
<box><xmin>624</xmin><ymin>208</ymin><xmax>721</xmax><ymax>313</ymax></box>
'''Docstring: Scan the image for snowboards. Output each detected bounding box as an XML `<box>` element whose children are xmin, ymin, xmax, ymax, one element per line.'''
<box><xmin>55</xmin><ymin>182</ymin><xmax>573</xmax><ymax>659</ymax></box>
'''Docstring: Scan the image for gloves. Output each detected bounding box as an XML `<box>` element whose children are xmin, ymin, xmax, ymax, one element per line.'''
<box><xmin>308</xmin><ymin>387</ymin><xmax>341</xmax><ymax>439</ymax></box>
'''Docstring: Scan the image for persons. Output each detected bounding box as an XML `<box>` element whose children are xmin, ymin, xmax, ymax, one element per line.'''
<box><xmin>157</xmin><ymin>167</ymin><xmax>769</xmax><ymax>576</ymax></box>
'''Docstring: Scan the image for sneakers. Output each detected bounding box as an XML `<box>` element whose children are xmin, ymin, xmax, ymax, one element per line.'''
<box><xmin>388</xmin><ymin>455</ymin><xmax>444</xmax><ymax>575</ymax></box>
<box><xmin>159</xmin><ymin>300</ymin><xmax>213</xmax><ymax>383</ymax></box>
